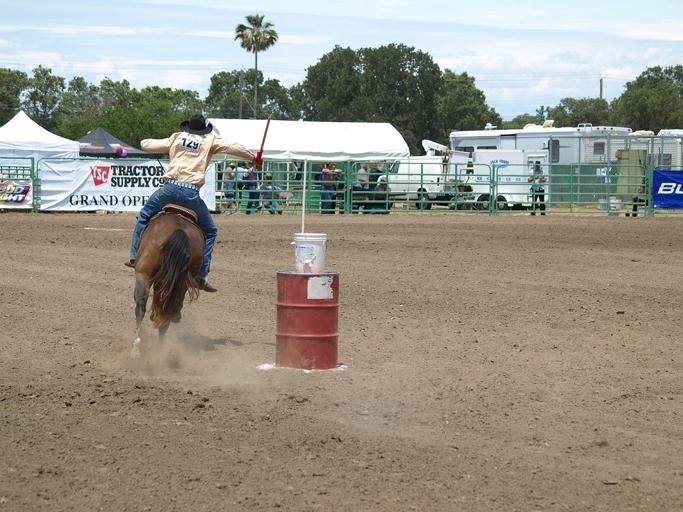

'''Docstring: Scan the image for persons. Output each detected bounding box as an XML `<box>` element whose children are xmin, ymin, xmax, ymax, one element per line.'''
<box><xmin>222</xmin><ymin>160</ymin><xmax>284</xmax><ymax>215</ymax></box>
<box><xmin>122</xmin><ymin>114</ymin><xmax>264</xmax><ymax>293</ymax></box>
<box><xmin>320</xmin><ymin>161</ymin><xmax>394</xmax><ymax>214</ymax></box>
<box><xmin>527</xmin><ymin>169</ymin><xmax>550</xmax><ymax>217</ymax></box>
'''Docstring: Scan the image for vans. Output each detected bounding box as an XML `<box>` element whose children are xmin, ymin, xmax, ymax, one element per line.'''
<box><xmin>376</xmin><ymin>149</ymin><xmax>469</xmax><ymax>208</ymax></box>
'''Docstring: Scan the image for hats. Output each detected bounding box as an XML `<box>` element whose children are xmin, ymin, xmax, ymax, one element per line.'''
<box><xmin>263</xmin><ymin>173</ymin><xmax>272</xmax><ymax>178</ymax></box>
<box><xmin>228</xmin><ymin>162</ymin><xmax>236</xmax><ymax>166</ymax></box>
<box><xmin>180</xmin><ymin>114</ymin><xmax>212</xmax><ymax>136</ymax></box>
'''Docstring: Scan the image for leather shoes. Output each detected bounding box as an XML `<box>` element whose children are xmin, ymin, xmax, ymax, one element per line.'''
<box><xmin>124</xmin><ymin>261</ymin><xmax>136</xmax><ymax>267</ymax></box>
<box><xmin>193</xmin><ymin>278</ymin><xmax>218</xmax><ymax>292</ymax></box>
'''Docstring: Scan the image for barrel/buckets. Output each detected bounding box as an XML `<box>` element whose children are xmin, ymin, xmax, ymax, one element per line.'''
<box><xmin>276</xmin><ymin>270</ymin><xmax>339</xmax><ymax>370</ymax></box>
<box><xmin>610</xmin><ymin>197</ymin><xmax>622</xmax><ymax>210</ymax></box>
<box><xmin>290</xmin><ymin>232</ymin><xmax>327</xmax><ymax>273</ymax></box>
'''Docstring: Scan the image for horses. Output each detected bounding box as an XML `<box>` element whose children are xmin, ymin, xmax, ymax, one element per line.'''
<box><xmin>130</xmin><ymin>211</ymin><xmax>204</xmax><ymax>360</ymax></box>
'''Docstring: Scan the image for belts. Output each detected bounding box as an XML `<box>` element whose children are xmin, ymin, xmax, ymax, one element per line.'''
<box><xmin>164</xmin><ymin>178</ymin><xmax>200</xmax><ymax>194</ymax></box>
